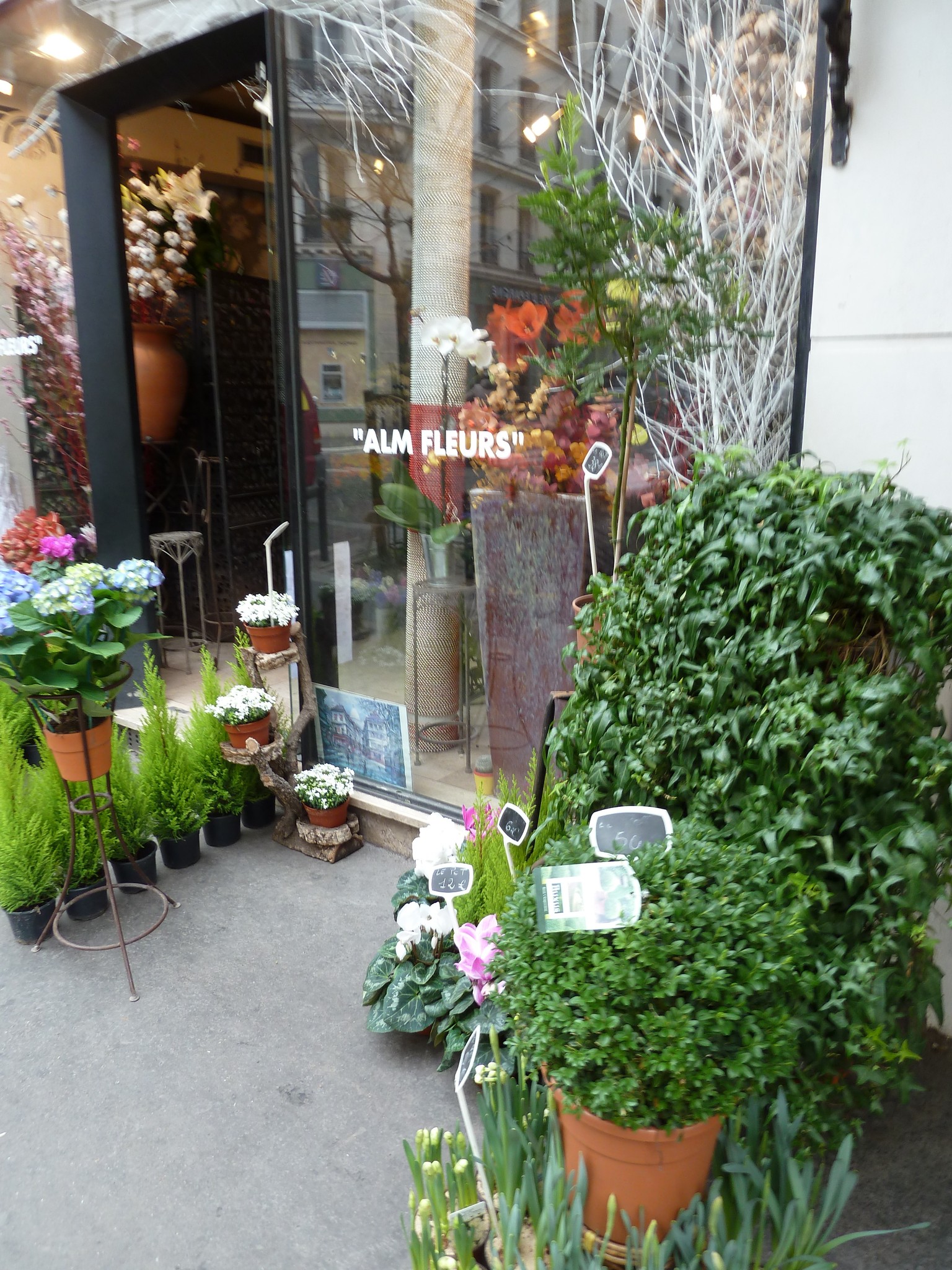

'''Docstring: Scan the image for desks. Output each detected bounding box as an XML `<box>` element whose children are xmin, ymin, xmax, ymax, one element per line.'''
<box><xmin>413</xmin><ymin>579</ymin><xmax>484</xmax><ymax>774</ymax></box>
<box><xmin>149</xmin><ymin>532</ymin><xmax>207</xmax><ymax>675</ymax></box>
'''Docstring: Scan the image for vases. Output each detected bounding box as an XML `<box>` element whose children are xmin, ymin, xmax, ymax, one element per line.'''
<box><xmin>302</xmin><ymin>795</ymin><xmax>350</xmax><ymax>828</ymax></box>
<box><xmin>243</xmin><ymin>621</ymin><xmax>291</xmax><ymax>654</ymax></box>
<box><xmin>223</xmin><ymin>713</ymin><xmax>271</xmax><ymax>749</ymax></box>
<box><xmin>132</xmin><ymin>323</ymin><xmax>187</xmax><ymax>444</ymax></box>
<box><xmin>43</xmin><ymin>708</ymin><xmax>111</xmax><ymax>782</ymax></box>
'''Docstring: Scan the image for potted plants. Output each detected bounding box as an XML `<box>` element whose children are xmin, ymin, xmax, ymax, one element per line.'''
<box><xmin>474</xmin><ymin>754</ymin><xmax>494</xmax><ymax>795</ymax></box>
<box><xmin>0</xmin><ymin>708</ymin><xmax>57</xmax><ymax>945</ymax></box>
<box><xmin>485</xmin><ymin>838</ymin><xmax>836</xmax><ymax>1248</ymax></box>
<box><xmin>136</xmin><ymin>642</ymin><xmax>202</xmax><ymax>869</ymax></box>
<box><xmin>241</xmin><ymin>765</ymin><xmax>276</xmax><ymax>829</ymax></box>
<box><xmin>33</xmin><ymin>711</ymin><xmax>107</xmax><ymax>923</ymax></box>
<box><xmin>373</xmin><ymin>482</ymin><xmax>473</xmax><ymax>586</ymax></box>
<box><xmin>99</xmin><ymin>723</ymin><xmax>157</xmax><ymax>895</ymax></box>
<box><xmin>184</xmin><ymin>692</ymin><xmax>241</xmax><ymax>848</ymax></box>
<box><xmin>571</xmin><ymin>573</ymin><xmax>616</xmax><ymax>665</ymax></box>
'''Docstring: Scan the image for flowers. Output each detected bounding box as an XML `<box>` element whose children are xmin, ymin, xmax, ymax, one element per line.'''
<box><xmin>390</xmin><ymin>801</ymin><xmax>510</xmax><ymax>1001</ymax></box>
<box><xmin>122</xmin><ymin>164</ymin><xmax>219</xmax><ymax>318</ymax></box>
<box><xmin>204</xmin><ymin>684</ymin><xmax>276</xmax><ymax>727</ymax></box>
<box><xmin>0</xmin><ymin>184</ymin><xmax>86</xmax><ymax>491</ymax></box>
<box><xmin>235</xmin><ymin>590</ymin><xmax>300</xmax><ymax>627</ymax></box>
<box><xmin>410</xmin><ymin>305</ymin><xmax>495</xmax><ymax>524</ymax></box>
<box><xmin>293</xmin><ymin>762</ymin><xmax>355</xmax><ymax>809</ymax></box>
<box><xmin>0</xmin><ymin>506</ymin><xmax>174</xmax><ymax>697</ymax></box>
